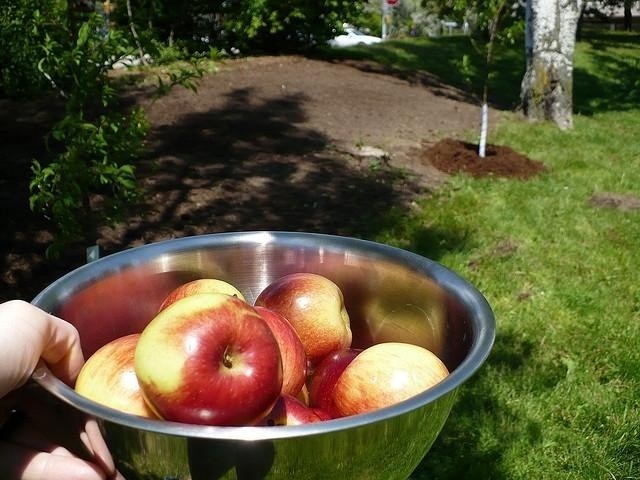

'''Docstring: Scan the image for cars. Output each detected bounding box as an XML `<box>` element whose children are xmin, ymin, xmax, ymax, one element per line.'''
<box><xmin>327</xmin><ymin>22</ymin><xmax>383</xmax><ymax>49</ymax></box>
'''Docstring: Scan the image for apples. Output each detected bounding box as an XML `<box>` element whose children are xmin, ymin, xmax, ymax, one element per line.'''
<box><xmin>73</xmin><ymin>272</ymin><xmax>451</xmax><ymax>426</ymax></box>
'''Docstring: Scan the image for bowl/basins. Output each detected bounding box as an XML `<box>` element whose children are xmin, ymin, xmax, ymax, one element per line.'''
<box><xmin>25</xmin><ymin>229</ymin><xmax>495</xmax><ymax>480</ymax></box>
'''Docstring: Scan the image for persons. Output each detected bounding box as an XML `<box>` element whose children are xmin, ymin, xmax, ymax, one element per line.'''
<box><xmin>0</xmin><ymin>299</ymin><xmax>124</xmax><ymax>479</ymax></box>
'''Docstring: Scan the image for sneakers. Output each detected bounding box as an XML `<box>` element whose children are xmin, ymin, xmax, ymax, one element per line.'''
<box><xmin>87</xmin><ymin>52</ymin><xmax>153</xmax><ymax>70</ymax></box>
